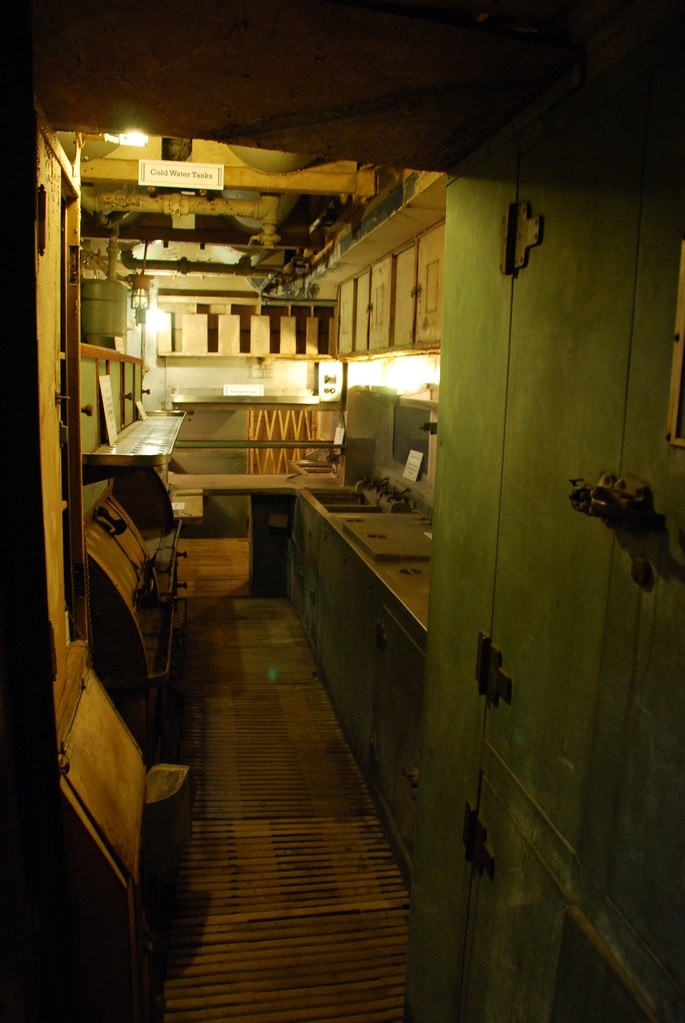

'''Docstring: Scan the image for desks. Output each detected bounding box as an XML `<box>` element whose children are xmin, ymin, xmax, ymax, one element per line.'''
<box><xmin>81</xmin><ymin>410</ymin><xmax>187</xmax><ymax>492</ymax></box>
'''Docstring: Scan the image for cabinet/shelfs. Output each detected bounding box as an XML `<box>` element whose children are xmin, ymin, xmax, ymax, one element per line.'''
<box><xmin>284</xmin><ymin>497</ymin><xmax>430</xmax><ymax>859</ymax></box>
<box><xmin>334</xmin><ymin>217</ymin><xmax>446</xmax><ymax>360</ymax></box>
<box><xmin>79</xmin><ymin>343</ymin><xmax>151</xmax><ymax>453</ymax></box>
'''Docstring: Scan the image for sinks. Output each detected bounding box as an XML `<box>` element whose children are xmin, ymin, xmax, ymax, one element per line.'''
<box><xmin>323</xmin><ymin>504</ymin><xmax>382</xmax><ymax>516</ymax></box>
<box><xmin>309</xmin><ymin>488</ymin><xmax>375</xmax><ymax>505</ymax></box>
<box><xmin>303</xmin><ymin>467</ymin><xmax>334</xmax><ymax>475</ymax></box>
<box><xmin>297</xmin><ymin>460</ymin><xmax>329</xmax><ymax>469</ymax></box>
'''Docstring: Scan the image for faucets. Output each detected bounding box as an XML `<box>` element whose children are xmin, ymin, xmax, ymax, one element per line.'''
<box><xmin>379</xmin><ymin>486</ymin><xmax>388</xmax><ymax>497</ymax></box>
<box><xmin>367</xmin><ymin>475</ymin><xmax>376</xmax><ymax>491</ymax></box>
<box><xmin>375</xmin><ymin>483</ymin><xmax>382</xmax><ymax>494</ymax></box>
<box><xmin>386</xmin><ymin>486</ymin><xmax>397</xmax><ymax>503</ymax></box>
<box><xmin>328</xmin><ymin>455</ymin><xmax>337</xmax><ymax>462</ymax></box>
<box><xmin>361</xmin><ymin>475</ymin><xmax>368</xmax><ymax>486</ymax></box>
<box><xmin>326</xmin><ymin>451</ymin><xmax>333</xmax><ymax>459</ymax></box>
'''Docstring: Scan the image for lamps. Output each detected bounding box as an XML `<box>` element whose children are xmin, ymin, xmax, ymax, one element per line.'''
<box><xmin>126</xmin><ymin>241</ymin><xmax>154</xmax><ymax>310</ymax></box>
